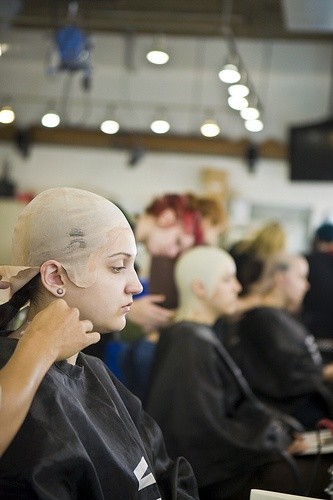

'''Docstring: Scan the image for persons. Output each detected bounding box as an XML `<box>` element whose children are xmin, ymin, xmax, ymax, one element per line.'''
<box><xmin>0</xmin><ymin>187</ymin><xmax>197</xmax><ymax>500</ymax></box>
<box><xmin>126</xmin><ymin>194</ymin><xmax>333</xmax><ymax>500</ymax></box>
<box><xmin>0</xmin><ymin>275</ymin><xmax>101</xmax><ymax>458</ymax></box>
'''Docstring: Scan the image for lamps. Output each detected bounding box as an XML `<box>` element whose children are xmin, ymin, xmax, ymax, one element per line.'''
<box><xmin>45</xmin><ymin>0</ymin><xmax>97</xmax><ymax>90</ymax></box>
<box><xmin>1</xmin><ymin>27</ymin><xmax>264</xmax><ymax>137</ymax></box>
